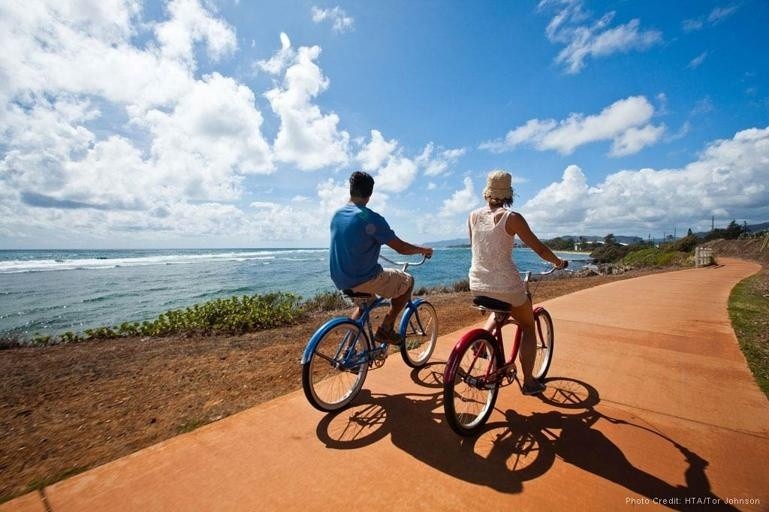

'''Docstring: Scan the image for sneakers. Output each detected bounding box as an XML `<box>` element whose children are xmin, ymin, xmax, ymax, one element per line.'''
<box><xmin>341</xmin><ymin>349</ymin><xmax>358</xmax><ymax>374</ymax></box>
<box><xmin>523</xmin><ymin>381</ymin><xmax>546</xmax><ymax>395</ymax></box>
<box><xmin>376</xmin><ymin>327</ymin><xmax>402</xmax><ymax>346</ymax></box>
<box><xmin>473</xmin><ymin>341</ymin><xmax>486</xmax><ymax>358</ymax></box>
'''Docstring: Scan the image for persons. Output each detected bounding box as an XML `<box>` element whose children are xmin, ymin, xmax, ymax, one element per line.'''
<box><xmin>466</xmin><ymin>169</ymin><xmax>568</xmax><ymax>397</ymax></box>
<box><xmin>329</xmin><ymin>170</ymin><xmax>434</xmax><ymax>364</ymax></box>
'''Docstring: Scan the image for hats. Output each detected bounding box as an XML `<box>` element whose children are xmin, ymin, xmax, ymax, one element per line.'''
<box><xmin>483</xmin><ymin>171</ymin><xmax>513</xmax><ymax>200</ymax></box>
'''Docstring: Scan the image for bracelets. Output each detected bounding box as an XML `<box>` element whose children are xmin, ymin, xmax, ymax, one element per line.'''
<box><xmin>556</xmin><ymin>256</ymin><xmax>564</xmax><ymax>267</ymax></box>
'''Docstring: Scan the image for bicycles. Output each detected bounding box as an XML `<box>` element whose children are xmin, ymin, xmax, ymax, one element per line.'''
<box><xmin>443</xmin><ymin>260</ymin><xmax>567</xmax><ymax>437</ymax></box>
<box><xmin>301</xmin><ymin>254</ymin><xmax>438</xmax><ymax>412</ymax></box>
